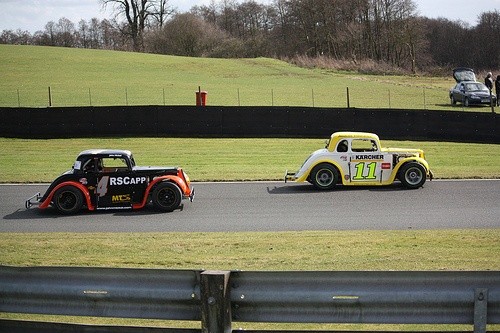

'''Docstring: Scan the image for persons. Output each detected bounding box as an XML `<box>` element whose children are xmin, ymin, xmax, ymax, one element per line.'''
<box><xmin>495</xmin><ymin>74</ymin><xmax>500</xmax><ymax>107</ymax></box>
<box><xmin>485</xmin><ymin>72</ymin><xmax>493</xmax><ymax>92</ymax></box>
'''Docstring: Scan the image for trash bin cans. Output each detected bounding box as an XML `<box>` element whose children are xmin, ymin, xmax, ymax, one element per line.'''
<box><xmin>195</xmin><ymin>91</ymin><xmax>207</xmax><ymax>106</ymax></box>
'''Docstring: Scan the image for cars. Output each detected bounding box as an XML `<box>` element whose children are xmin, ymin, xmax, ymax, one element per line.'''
<box><xmin>449</xmin><ymin>67</ymin><xmax>497</xmax><ymax>107</ymax></box>
<box><xmin>25</xmin><ymin>148</ymin><xmax>196</xmax><ymax>215</ymax></box>
<box><xmin>284</xmin><ymin>131</ymin><xmax>434</xmax><ymax>190</ymax></box>
